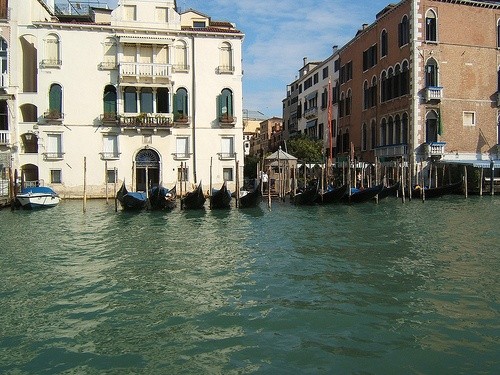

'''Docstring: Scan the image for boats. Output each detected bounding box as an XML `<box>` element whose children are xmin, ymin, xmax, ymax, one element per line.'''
<box><xmin>16</xmin><ymin>186</ymin><xmax>60</xmax><ymax>209</ymax></box>
<box><xmin>386</xmin><ymin>178</ymin><xmax>466</xmax><ymax>199</ymax></box>
<box><xmin>117</xmin><ymin>178</ymin><xmax>265</xmax><ymax>210</ymax></box>
<box><xmin>290</xmin><ymin>176</ymin><xmax>401</xmax><ymax>206</ymax></box>
